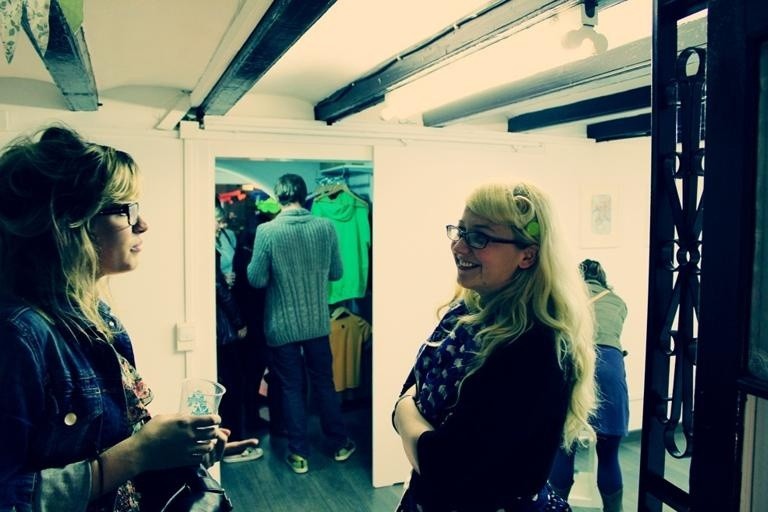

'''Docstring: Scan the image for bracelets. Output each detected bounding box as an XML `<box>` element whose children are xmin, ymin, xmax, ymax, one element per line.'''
<box><xmin>395</xmin><ymin>394</ymin><xmax>415</xmax><ymax>407</ymax></box>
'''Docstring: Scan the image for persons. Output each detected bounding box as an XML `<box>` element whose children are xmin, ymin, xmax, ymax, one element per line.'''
<box><xmin>546</xmin><ymin>258</ymin><xmax>630</xmax><ymax>511</ymax></box>
<box><xmin>390</xmin><ymin>181</ymin><xmax>602</xmax><ymax>512</ymax></box>
<box><xmin>247</xmin><ymin>173</ymin><xmax>357</xmax><ymax>473</ymax></box>
<box><xmin>215</xmin><ymin>195</ymin><xmax>264</xmax><ymax>462</ymax></box>
<box><xmin>0</xmin><ymin>119</ymin><xmax>259</xmax><ymax>512</ymax></box>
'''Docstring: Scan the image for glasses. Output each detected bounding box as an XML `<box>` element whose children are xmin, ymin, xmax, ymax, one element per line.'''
<box><xmin>447</xmin><ymin>224</ymin><xmax>530</xmax><ymax>248</ymax></box>
<box><xmin>99</xmin><ymin>201</ymin><xmax>139</xmax><ymax>226</ymax></box>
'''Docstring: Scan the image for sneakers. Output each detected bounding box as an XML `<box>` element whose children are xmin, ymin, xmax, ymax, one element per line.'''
<box><xmin>335</xmin><ymin>440</ymin><xmax>356</xmax><ymax>461</ymax></box>
<box><xmin>224</xmin><ymin>448</ymin><xmax>262</xmax><ymax>463</ymax></box>
<box><xmin>285</xmin><ymin>452</ymin><xmax>309</xmax><ymax>474</ymax></box>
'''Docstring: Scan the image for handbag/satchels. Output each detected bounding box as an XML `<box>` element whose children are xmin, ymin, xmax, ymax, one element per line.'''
<box><xmin>513</xmin><ymin>486</ymin><xmax>573</xmax><ymax>512</ymax></box>
<box><xmin>132</xmin><ymin>465</ymin><xmax>233</xmax><ymax>511</ymax></box>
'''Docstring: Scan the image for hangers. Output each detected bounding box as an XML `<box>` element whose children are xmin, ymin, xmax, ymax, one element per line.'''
<box><xmin>317</xmin><ymin>182</ymin><xmax>358</xmax><ymax>200</ymax></box>
<box><xmin>330</xmin><ymin>306</ymin><xmax>352</xmax><ymax>321</ymax></box>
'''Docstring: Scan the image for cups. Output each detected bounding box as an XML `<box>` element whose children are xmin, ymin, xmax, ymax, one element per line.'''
<box><xmin>180</xmin><ymin>377</ymin><xmax>227</xmax><ymax>443</ymax></box>
